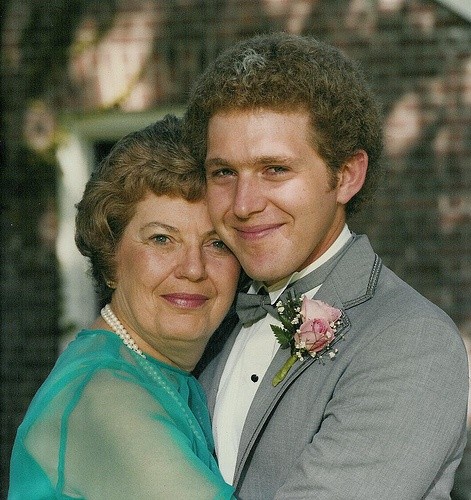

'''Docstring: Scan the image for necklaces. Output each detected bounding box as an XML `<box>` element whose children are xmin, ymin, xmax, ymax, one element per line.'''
<box><xmin>100</xmin><ymin>303</ymin><xmax>209</xmax><ymax>456</ymax></box>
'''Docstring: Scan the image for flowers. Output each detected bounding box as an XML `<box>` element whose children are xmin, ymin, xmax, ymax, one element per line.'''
<box><xmin>271</xmin><ymin>290</ymin><xmax>344</xmax><ymax>387</ymax></box>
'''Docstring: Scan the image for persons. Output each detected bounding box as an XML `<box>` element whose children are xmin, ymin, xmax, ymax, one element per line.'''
<box><xmin>183</xmin><ymin>32</ymin><xmax>468</xmax><ymax>500</ymax></box>
<box><xmin>7</xmin><ymin>115</ymin><xmax>237</xmax><ymax>500</ymax></box>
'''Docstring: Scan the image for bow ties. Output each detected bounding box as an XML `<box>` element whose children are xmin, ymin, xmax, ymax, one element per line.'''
<box><xmin>236</xmin><ymin>231</ymin><xmax>358</xmax><ymax>328</ymax></box>
